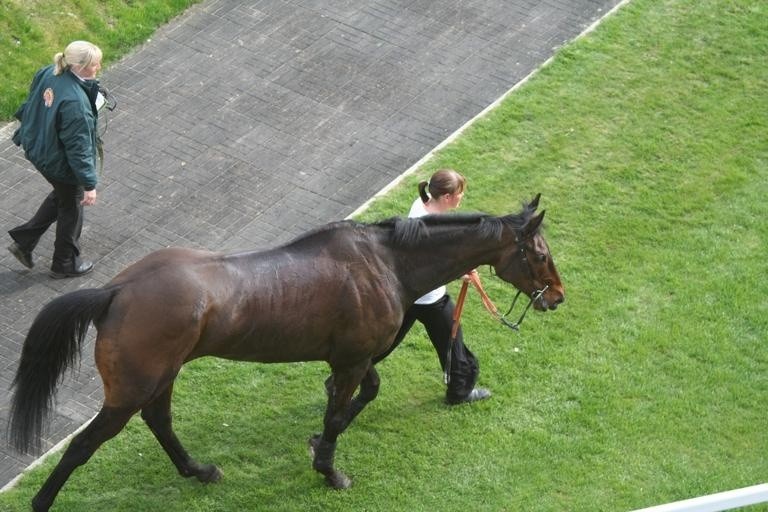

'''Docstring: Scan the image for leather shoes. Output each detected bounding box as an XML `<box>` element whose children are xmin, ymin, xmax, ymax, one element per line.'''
<box><xmin>444</xmin><ymin>388</ymin><xmax>493</xmax><ymax>405</ymax></box>
<box><xmin>7</xmin><ymin>239</ymin><xmax>36</xmax><ymax>269</ymax></box>
<box><xmin>48</xmin><ymin>260</ymin><xmax>95</xmax><ymax>280</ymax></box>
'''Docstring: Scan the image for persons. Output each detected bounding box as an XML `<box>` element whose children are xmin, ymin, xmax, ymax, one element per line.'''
<box><xmin>7</xmin><ymin>38</ymin><xmax>106</xmax><ymax>278</ymax></box>
<box><xmin>322</xmin><ymin>166</ymin><xmax>494</xmax><ymax>407</ymax></box>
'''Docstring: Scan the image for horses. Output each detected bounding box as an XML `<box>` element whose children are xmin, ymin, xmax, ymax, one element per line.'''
<box><xmin>1</xmin><ymin>192</ymin><xmax>566</xmax><ymax>512</ymax></box>
